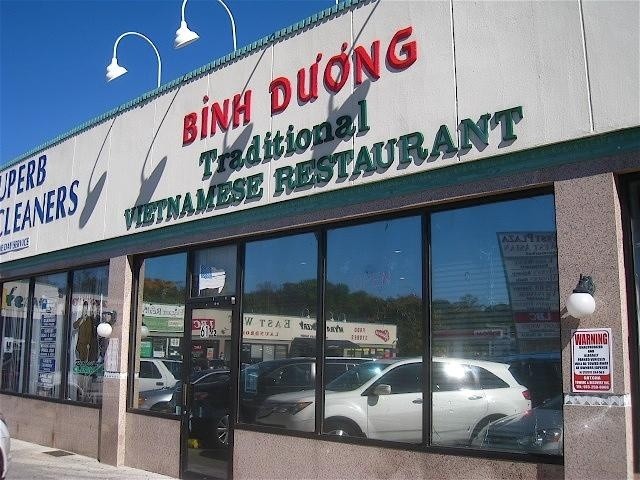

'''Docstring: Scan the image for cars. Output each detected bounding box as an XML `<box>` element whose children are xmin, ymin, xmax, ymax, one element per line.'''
<box><xmin>0</xmin><ymin>411</ymin><xmax>12</xmax><ymax>480</ymax></box>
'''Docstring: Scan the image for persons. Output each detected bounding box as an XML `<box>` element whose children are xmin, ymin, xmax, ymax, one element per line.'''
<box><xmin>73</xmin><ymin>300</ymin><xmax>100</xmax><ymax>362</ymax></box>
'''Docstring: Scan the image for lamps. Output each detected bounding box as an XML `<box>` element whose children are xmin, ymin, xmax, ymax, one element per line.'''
<box><xmin>172</xmin><ymin>1</ymin><xmax>240</xmax><ymax>52</ymax></box>
<box><xmin>142</xmin><ymin>313</ymin><xmax>150</xmax><ymax>342</ymax></box>
<box><xmin>94</xmin><ymin>291</ymin><xmax>118</xmax><ymax>339</ymax></box>
<box><xmin>104</xmin><ymin>29</ymin><xmax>164</xmax><ymax>96</ymax></box>
<box><xmin>564</xmin><ymin>274</ymin><xmax>598</xmax><ymax>324</ymax></box>
